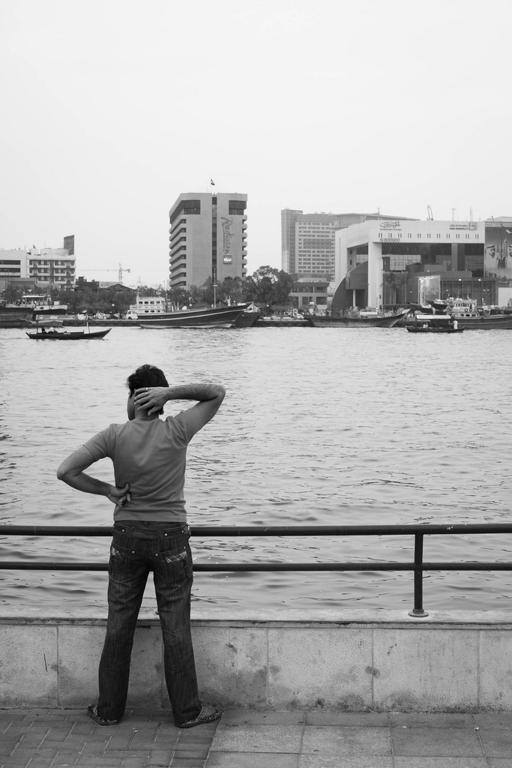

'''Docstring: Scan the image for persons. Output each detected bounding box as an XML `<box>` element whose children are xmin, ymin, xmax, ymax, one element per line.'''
<box><xmin>451</xmin><ymin>318</ymin><xmax>458</xmax><ymax>329</ymax></box>
<box><xmin>56</xmin><ymin>362</ymin><xmax>227</xmax><ymax>729</ymax></box>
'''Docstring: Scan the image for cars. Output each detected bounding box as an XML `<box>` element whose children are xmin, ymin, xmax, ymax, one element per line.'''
<box><xmin>271</xmin><ymin>308</ymin><xmax>305</xmax><ymax>322</ymax></box>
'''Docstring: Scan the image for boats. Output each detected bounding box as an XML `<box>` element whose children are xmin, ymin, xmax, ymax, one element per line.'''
<box><xmin>406</xmin><ymin>326</ymin><xmax>466</xmax><ymax>333</ymax></box>
<box><xmin>25</xmin><ymin>327</ymin><xmax>112</xmax><ymax>339</ymax></box>
<box><xmin>410</xmin><ymin>303</ymin><xmax>512</xmax><ymax>330</ymax></box>
<box><xmin>303</xmin><ymin>309</ymin><xmax>410</xmax><ymax>328</ymax></box>
<box><xmin>136</xmin><ymin>300</ymin><xmax>266</xmax><ymax>328</ymax></box>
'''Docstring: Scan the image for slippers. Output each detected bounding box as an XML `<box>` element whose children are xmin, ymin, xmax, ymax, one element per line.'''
<box><xmin>87</xmin><ymin>703</ymin><xmax>122</xmax><ymax>726</ymax></box>
<box><xmin>176</xmin><ymin>702</ymin><xmax>224</xmax><ymax>729</ymax></box>
